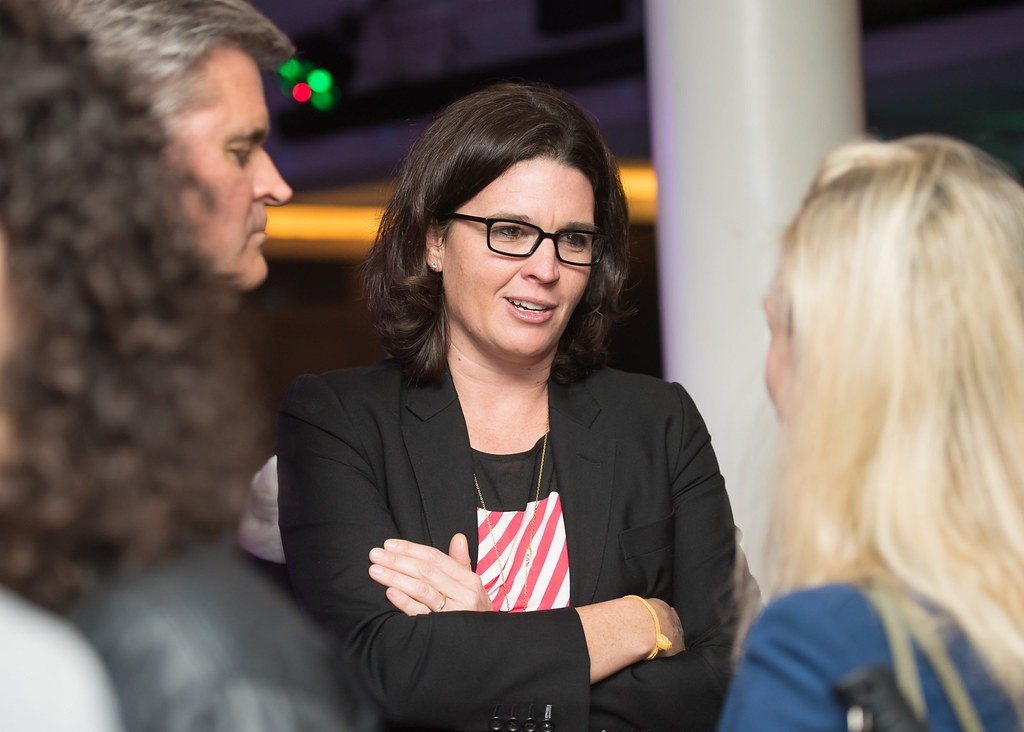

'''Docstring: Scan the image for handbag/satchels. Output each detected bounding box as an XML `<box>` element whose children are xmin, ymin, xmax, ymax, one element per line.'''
<box><xmin>829</xmin><ymin>580</ymin><xmax>985</xmax><ymax>732</ymax></box>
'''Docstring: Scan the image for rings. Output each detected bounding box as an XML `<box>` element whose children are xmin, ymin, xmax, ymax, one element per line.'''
<box><xmin>434</xmin><ymin>594</ymin><xmax>448</xmax><ymax>612</ymax></box>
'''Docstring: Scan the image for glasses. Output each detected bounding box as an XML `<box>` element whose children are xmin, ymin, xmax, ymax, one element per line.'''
<box><xmin>451</xmin><ymin>207</ymin><xmax>611</xmax><ymax>266</ymax></box>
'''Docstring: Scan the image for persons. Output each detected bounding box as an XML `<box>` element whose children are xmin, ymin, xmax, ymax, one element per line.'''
<box><xmin>0</xmin><ymin>0</ymin><xmax>374</xmax><ymax>732</ymax></box>
<box><xmin>715</xmin><ymin>133</ymin><xmax>1024</xmax><ymax>732</ymax></box>
<box><xmin>276</xmin><ymin>83</ymin><xmax>763</xmax><ymax>732</ymax></box>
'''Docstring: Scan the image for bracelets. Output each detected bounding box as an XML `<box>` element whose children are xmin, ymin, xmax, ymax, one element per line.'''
<box><xmin>622</xmin><ymin>594</ymin><xmax>674</xmax><ymax>662</ymax></box>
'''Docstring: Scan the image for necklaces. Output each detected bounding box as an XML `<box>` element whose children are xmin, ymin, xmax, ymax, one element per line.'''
<box><xmin>469</xmin><ymin>425</ymin><xmax>550</xmax><ymax>613</ymax></box>
